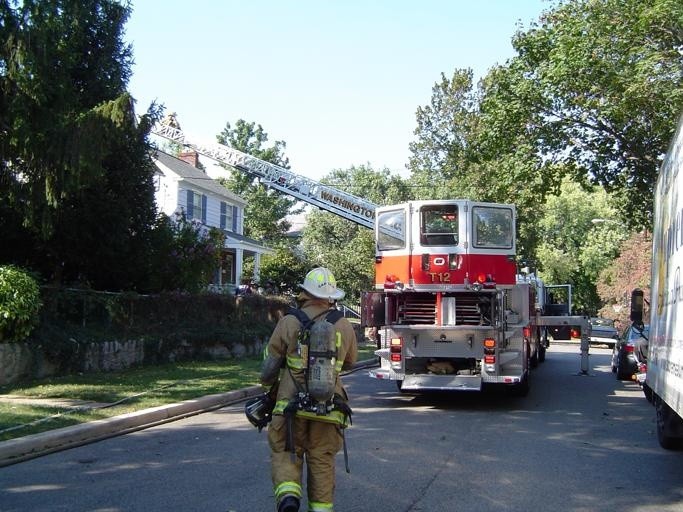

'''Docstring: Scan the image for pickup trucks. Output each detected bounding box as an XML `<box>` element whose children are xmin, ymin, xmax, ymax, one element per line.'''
<box><xmin>587</xmin><ymin>317</ymin><xmax>619</xmax><ymax>349</ymax></box>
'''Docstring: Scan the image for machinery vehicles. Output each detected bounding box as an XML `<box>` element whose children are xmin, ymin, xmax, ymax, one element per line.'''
<box><xmin>135</xmin><ymin>113</ymin><xmax>592</xmax><ymax>393</ymax></box>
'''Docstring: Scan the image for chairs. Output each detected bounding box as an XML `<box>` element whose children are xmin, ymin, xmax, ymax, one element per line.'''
<box><xmin>426</xmin><ymin>227</ymin><xmax>456</xmax><ymax>245</ymax></box>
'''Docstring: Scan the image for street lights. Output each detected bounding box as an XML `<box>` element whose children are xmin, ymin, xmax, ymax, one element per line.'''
<box><xmin>590</xmin><ymin>218</ymin><xmax>629</xmax><ymax>232</ymax></box>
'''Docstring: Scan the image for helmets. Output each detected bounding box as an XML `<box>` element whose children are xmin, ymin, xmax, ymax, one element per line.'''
<box><xmin>298</xmin><ymin>267</ymin><xmax>345</xmax><ymax>300</ymax></box>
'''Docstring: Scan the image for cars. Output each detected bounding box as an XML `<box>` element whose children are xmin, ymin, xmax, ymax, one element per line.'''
<box><xmin>610</xmin><ymin>324</ymin><xmax>650</xmax><ymax>381</ymax></box>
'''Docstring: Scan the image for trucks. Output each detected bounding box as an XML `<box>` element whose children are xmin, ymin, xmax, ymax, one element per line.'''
<box><xmin>643</xmin><ymin>113</ymin><xmax>682</xmax><ymax>452</ymax></box>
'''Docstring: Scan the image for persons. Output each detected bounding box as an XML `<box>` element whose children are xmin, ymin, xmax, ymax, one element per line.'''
<box><xmin>260</xmin><ymin>267</ymin><xmax>358</xmax><ymax>511</ymax></box>
